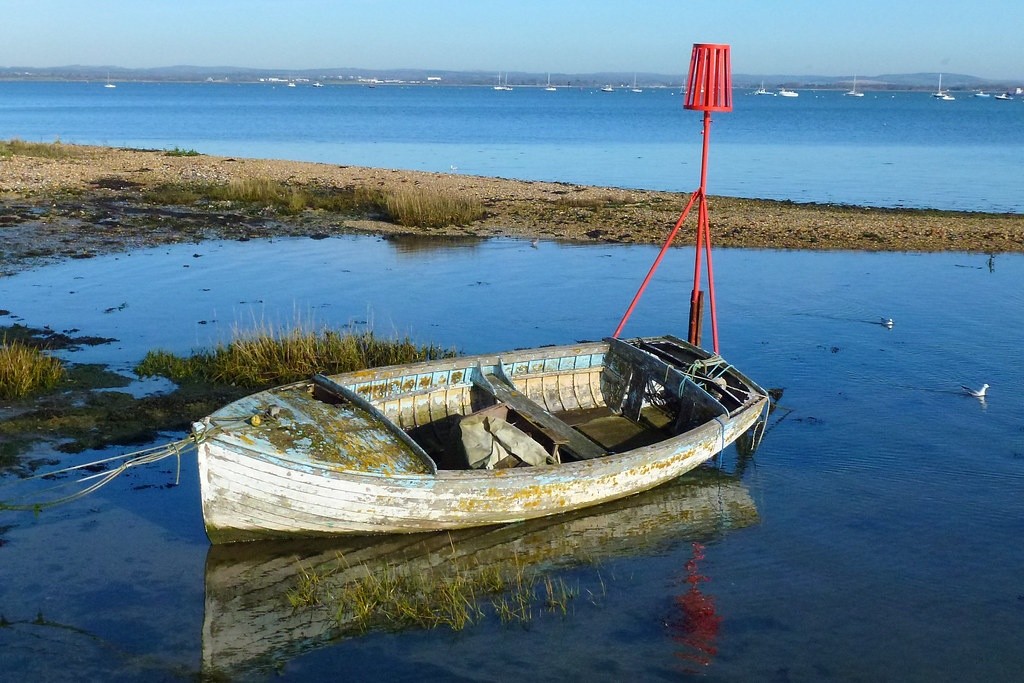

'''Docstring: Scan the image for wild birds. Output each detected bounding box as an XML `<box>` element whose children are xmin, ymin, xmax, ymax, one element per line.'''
<box><xmin>957</xmin><ymin>382</ymin><xmax>992</xmax><ymax>398</ymax></box>
<box><xmin>879</xmin><ymin>315</ymin><xmax>893</xmax><ymax>326</ymax></box>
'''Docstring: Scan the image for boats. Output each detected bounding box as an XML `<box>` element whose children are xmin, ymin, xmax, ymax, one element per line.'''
<box><xmin>973</xmin><ymin>90</ymin><xmax>989</xmax><ymax>97</ymax></box>
<box><xmin>287</xmin><ymin>82</ymin><xmax>297</xmax><ymax>87</ymax></box>
<box><xmin>201</xmin><ymin>334</ymin><xmax>773</xmax><ymax>536</ymax></box>
<box><xmin>993</xmin><ymin>90</ymin><xmax>1014</xmax><ymax>101</ymax></box>
<box><xmin>314</xmin><ymin>82</ymin><xmax>324</xmax><ymax>88</ymax></box>
<box><xmin>778</xmin><ymin>87</ymin><xmax>799</xmax><ymax>98</ymax></box>
<box><xmin>200</xmin><ymin>463</ymin><xmax>762</xmax><ymax>683</ymax></box>
<box><xmin>598</xmin><ymin>82</ymin><xmax>617</xmax><ymax>93</ymax></box>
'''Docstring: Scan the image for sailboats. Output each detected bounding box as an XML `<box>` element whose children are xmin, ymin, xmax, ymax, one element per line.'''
<box><xmin>493</xmin><ymin>72</ymin><xmax>512</xmax><ymax>92</ymax></box>
<box><xmin>104</xmin><ymin>72</ymin><xmax>118</xmax><ymax>89</ymax></box>
<box><xmin>933</xmin><ymin>72</ymin><xmax>954</xmax><ymax>101</ymax></box>
<box><xmin>546</xmin><ymin>72</ymin><xmax>557</xmax><ymax>93</ymax></box>
<box><xmin>847</xmin><ymin>74</ymin><xmax>864</xmax><ymax>98</ymax></box>
<box><xmin>632</xmin><ymin>74</ymin><xmax>646</xmax><ymax>94</ymax></box>
<box><xmin>758</xmin><ymin>85</ymin><xmax>768</xmax><ymax>97</ymax></box>
